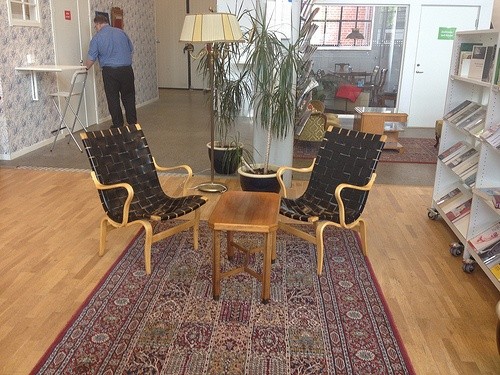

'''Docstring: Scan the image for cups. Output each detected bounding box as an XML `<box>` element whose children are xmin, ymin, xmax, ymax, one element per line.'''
<box><xmin>348</xmin><ymin>66</ymin><xmax>352</xmax><ymax>72</ymax></box>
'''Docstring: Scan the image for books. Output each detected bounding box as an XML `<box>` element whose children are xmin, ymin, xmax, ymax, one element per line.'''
<box><xmin>436</xmin><ymin>41</ymin><xmax>500</xmax><ymax>284</ymax></box>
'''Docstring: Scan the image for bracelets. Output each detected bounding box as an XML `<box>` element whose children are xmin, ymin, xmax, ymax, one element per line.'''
<box><xmin>86</xmin><ymin>68</ymin><xmax>88</xmax><ymax>70</ymax></box>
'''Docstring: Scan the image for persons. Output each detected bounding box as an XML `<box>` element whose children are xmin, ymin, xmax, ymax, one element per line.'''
<box><xmin>85</xmin><ymin>15</ymin><xmax>137</xmax><ymax>128</ymax></box>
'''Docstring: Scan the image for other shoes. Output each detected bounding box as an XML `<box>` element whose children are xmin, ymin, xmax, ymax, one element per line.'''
<box><xmin>110</xmin><ymin>126</ymin><xmax>122</xmax><ymax>128</ymax></box>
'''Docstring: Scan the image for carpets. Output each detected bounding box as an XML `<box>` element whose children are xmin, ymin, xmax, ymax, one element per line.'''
<box><xmin>29</xmin><ymin>218</ymin><xmax>417</xmax><ymax>375</ymax></box>
<box><xmin>293</xmin><ymin>136</ymin><xmax>437</xmax><ymax>163</ymax></box>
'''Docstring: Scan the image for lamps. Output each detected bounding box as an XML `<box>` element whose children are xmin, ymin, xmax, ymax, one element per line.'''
<box><xmin>345</xmin><ymin>6</ymin><xmax>365</xmax><ymax>39</ymax></box>
<box><xmin>178</xmin><ymin>11</ymin><xmax>244</xmax><ymax>193</ymax></box>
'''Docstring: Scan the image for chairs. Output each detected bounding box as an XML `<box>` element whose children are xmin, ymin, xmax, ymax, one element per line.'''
<box><xmin>326</xmin><ymin>64</ymin><xmax>398</xmax><ymax>108</ymax></box>
<box><xmin>272</xmin><ymin>126</ymin><xmax>388</xmax><ymax>275</ymax></box>
<box><xmin>293</xmin><ymin>100</ymin><xmax>326</xmax><ymax>151</ymax></box>
<box><xmin>80</xmin><ymin>123</ymin><xmax>206</xmax><ymax>272</ymax></box>
<box><xmin>49</xmin><ymin>70</ymin><xmax>90</xmax><ymax>153</ymax></box>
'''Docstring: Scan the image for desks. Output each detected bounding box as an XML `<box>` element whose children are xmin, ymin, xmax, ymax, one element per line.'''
<box><xmin>205</xmin><ymin>189</ymin><xmax>280</xmax><ymax>305</ymax></box>
<box><xmin>352</xmin><ymin>106</ymin><xmax>408</xmax><ymax>153</ymax></box>
<box><xmin>14</xmin><ymin>65</ymin><xmax>87</xmax><ymax>100</ymax></box>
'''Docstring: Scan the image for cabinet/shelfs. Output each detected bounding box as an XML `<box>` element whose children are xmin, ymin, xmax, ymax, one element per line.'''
<box><xmin>426</xmin><ymin>27</ymin><xmax>500</xmax><ymax>298</ymax></box>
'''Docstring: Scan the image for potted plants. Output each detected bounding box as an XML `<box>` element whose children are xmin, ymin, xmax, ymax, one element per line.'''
<box><xmin>201</xmin><ymin>1</ymin><xmax>321</xmax><ymax>193</ymax></box>
